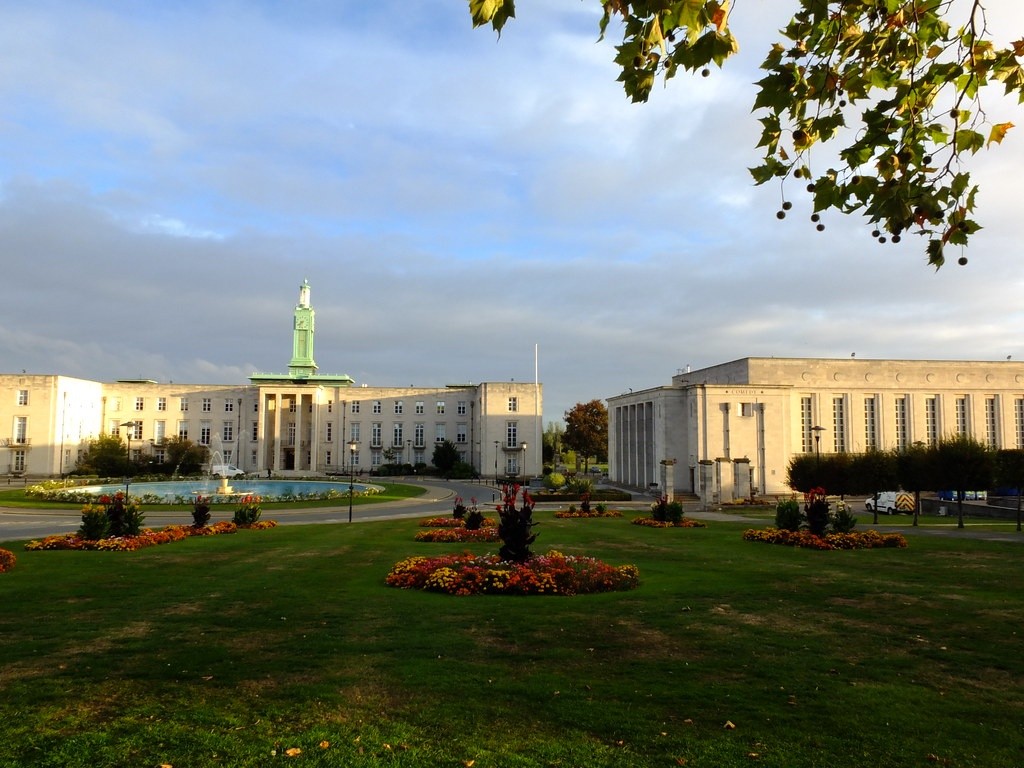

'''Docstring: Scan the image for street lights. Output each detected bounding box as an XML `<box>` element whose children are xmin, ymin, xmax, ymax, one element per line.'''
<box><xmin>120</xmin><ymin>421</ymin><xmax>140</xmax><ymax>507</ymax></box>
<box><xmin>346</xmin><ymin>441</ymin><xmax>358</xmax><ymax>523</ymax></box>
<box><xmin>406</xmin><ymin>439</ymin><xmax>412</xmax><ymax>461</ymax></box>
<box><xmin>520</xmin><ymin>441</ymin><xmax>528</xmax><ymax>488</ymax></box>
<box><xmin>810</xmin><ymin>425</ymin><xmax>828</xmax><ymax>462</ymax></box>
<box><xmin>493</xmin><ymin>440</ymin><xmax>501</xmax><ymax>484</ymax></box>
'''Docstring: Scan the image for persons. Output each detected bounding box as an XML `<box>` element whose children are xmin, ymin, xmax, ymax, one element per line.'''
<box><xmin>266</xmin><ymin>468</ymin><xmax>272</xmax><ymax>480</ymax></box>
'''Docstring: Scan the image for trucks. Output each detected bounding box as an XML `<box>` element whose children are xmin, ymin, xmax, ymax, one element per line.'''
<box><xmin>213</xmin><ymin>465</ymin><xmax>245</xmax><ymax>480</ymax></box>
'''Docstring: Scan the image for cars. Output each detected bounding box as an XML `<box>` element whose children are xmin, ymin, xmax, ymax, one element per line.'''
<box><xmin>951</xmin><ymin>493</ymin><xmax>984</xmax><ymax>501</ymax></box>
<box><xmin>590</xmin><ymin>467</ymin><xmax>601</xmax><ymax>473</ymax></box>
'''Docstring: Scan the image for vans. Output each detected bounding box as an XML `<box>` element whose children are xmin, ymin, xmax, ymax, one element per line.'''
<box><xmin>864</xmin><ymin>492</ymin><xmax>915</xmax><ymax>516</ymax></box>
